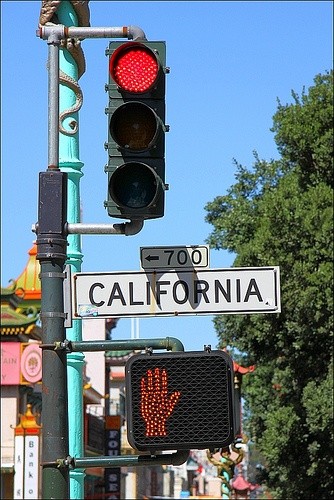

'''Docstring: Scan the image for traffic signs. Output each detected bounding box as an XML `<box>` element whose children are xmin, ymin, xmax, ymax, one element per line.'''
<box><xmin>72</xmin><ymin>247</ymin><xmax>282</xmax><ymax>322</ymax></box>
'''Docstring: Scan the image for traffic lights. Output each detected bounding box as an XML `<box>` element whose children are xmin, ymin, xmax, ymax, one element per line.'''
<box><xmin>126</xmin><ymin>350</ymin><xmax>235</xmax><ymax>453</ymax></box>
<box><xmin>105</xmin><ymin>40</ymin><xmax>170</xmax><ymax>221</ymax></box>
